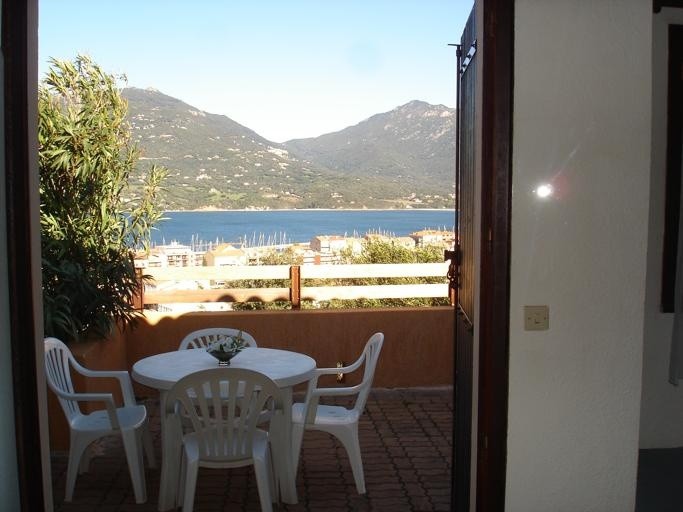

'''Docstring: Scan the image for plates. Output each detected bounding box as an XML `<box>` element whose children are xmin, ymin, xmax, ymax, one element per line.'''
<box><xmin>207</xmin><ymin>349</ymin><xmax>242</xmax><ymax>365</ymax></box>
